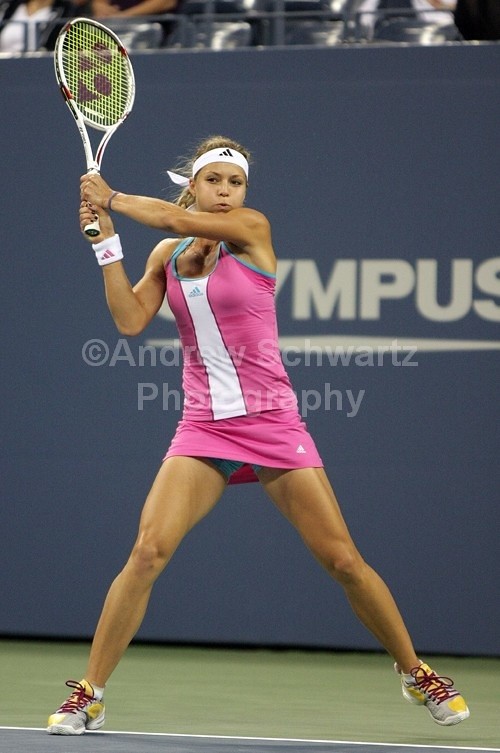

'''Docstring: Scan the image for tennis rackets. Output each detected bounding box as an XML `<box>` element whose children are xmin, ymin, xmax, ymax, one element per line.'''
<box><xmin>52</xmin><ymin>17</ymin><xmax>136</xmax><ymax>238</ymax></box>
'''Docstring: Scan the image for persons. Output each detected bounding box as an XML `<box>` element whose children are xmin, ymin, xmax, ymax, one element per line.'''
<box><xmin>1</xmin><ymin>0</ymin><xmax>500</xmax><ymax>52</ymax></box>
<box><xmin>45</xmin><ymin>136</ymin><xmax>472</xmax><ymax>736</ymax></box>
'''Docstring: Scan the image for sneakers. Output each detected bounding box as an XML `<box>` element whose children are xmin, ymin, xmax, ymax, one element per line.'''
<box><xmin>395</xmin><ymin>658</ymin><xmax>470</xmax><ymax>726</ymax></box>
<box><xmin>46</xmin><ymin>678</ymin><xmax>105</xmax><ymax>736</ymax></box>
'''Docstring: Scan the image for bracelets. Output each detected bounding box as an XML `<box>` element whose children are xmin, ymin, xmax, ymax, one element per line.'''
<box><xmin>108</xmin><ymin>191</ymin><xmax>121</xmax><ymax>210</ymax></box>
<box><xmin>93</xmin><ymin>233</ymin><xmax>126</xmax><ymax>266</ymax></box>
